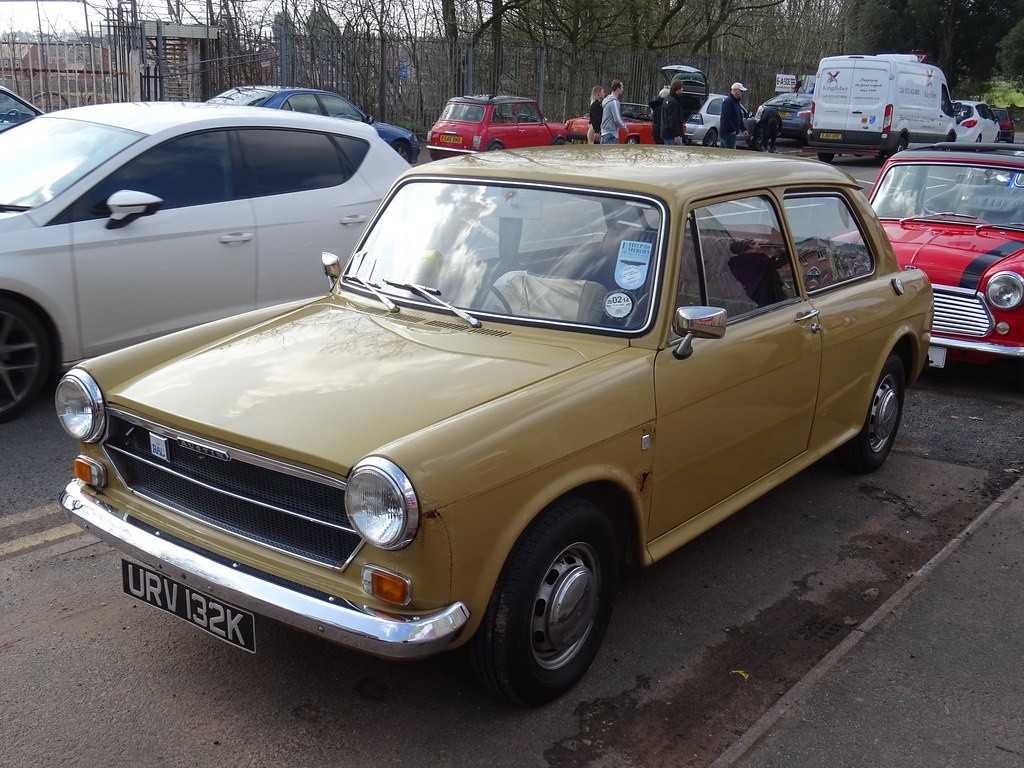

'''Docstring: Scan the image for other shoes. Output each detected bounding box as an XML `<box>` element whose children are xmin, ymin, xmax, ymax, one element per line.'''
<box><xmin>763</xmin><ymin>148</ymin><xmax>769</xmax><ymax>152</ymax></box>
<box><xmin>769</xmin><ymin>148</ymin><xmax>778</xmax><ymax>153</ymax></box>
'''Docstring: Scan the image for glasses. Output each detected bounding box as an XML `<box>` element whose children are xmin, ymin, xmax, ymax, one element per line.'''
<box><xmin>740</xmin><ymin>90</ymin><xmax>745</xmax><ymax>92</ymax></box>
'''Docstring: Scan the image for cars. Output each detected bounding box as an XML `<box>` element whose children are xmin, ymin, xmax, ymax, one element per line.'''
<box><xmin>0</xmin><ymin>86</ymin><xmax>48</xmax><ymax>135</ymax></box>
<box><xmin>0</xmin><ymin>102</ymin><xmax>415</xmax><ymax>425</ymax></box>
<box><xmin>755</xmin><ymin>93</ymin><xmax>815</xmax><ymax>145</ymax></box>
<box><xmin>950</xmin><ymin>100</ymin><xmax>1002</xmax><ymax>154</ymax></box>
<box><xmin>802</xmin><ymin>142</ymin><xmax>1024</xmax><ymax>368</ymax></box>
<box><xmin>653</xmin><ymin>65</ymin><xmax>764</xmax><ymax>150</ymax></box>
<box><xmin>564</xmin><ymin>103</ymin><xmax>687</xmax><ymax>144</ymax></box>
<box><xmin>57</xmin><ymin>144</ymin><xmax>934</xmax><ymax>710</ymax></box>
<box><xmin>205</xmin><ymin>84</ymin><xmax>421</xmax><ymax>176</ymax></box>
<box><xmin>990</xmin><ymin>108</ymin><xmax>1020</xmax><ymax>144</ymax></box>
<box><xmin>427</xmin><ymin>95</ymin><xmax>565</xmax><ymax>162</ymax></box>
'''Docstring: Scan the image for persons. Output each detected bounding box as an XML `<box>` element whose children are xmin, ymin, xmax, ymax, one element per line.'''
<box><xmin>728</xmin><ymin>240</ymin><xmax>790</xmax><ymax>307</ymax></box>
<box><xmin>720</xmin><ymin>83</ymin><xmax>748</xmax><ymax>149</ymax></box>
<box><xmin>750</xmin><ymin>108</ymin><xmax>783</xmax><ymax>153</ymax></box>
<box><xmin>587</xmin><ymin>86</ymin><xmax>605</xmax><ymax>144</ymax></box>
<box><xmin>648</xmin><ymin>80</ymin><xmax>684</xmax><ymax>145</ymax></box>
<box><xmin>601</xmin><ymin>80</ymin><xmax>629</xmax><ymax>144</ymax></box>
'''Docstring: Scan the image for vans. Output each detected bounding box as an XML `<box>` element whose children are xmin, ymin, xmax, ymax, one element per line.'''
<box><xmin>808</xmin><ymin>55</ymin><xmax>960</xmax><ymax>165</ymax></box>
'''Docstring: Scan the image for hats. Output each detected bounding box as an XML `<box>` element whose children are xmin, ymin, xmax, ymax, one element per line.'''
<box><xmin>659</xmin><ymin>88</ymin><xmax>670</xmax><ymax>98</ymax></box>
<box><xmin>729</xmin><ymin>238</ymin><xmax>753</xmax><ymax>254</ymax></box>
<box><xmin>731</xmin><ymin>82</ymin><xmax>748</xmax><ymax>91</ymax></box>
<box><xmin>670</xmin><ymin>79</ymin><xmax>682</xmax><ymax>90</ymax></box>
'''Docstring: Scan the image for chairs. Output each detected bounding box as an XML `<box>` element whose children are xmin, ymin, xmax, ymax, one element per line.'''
<box><xmin>622</xmin><ymin>289</ymin><xmax>760</xmax><ymax>331</ymax></box>
<box><xmin>486</xmin><ymin>273</ymin><xmax>607</xmax><ymax>326</ymax></box>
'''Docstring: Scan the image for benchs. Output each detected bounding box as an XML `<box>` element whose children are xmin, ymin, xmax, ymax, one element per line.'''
<box><xmin>579</xmin><ymin>227</ymin><xmax>844</xmax><ymax>319</ymax></box>
